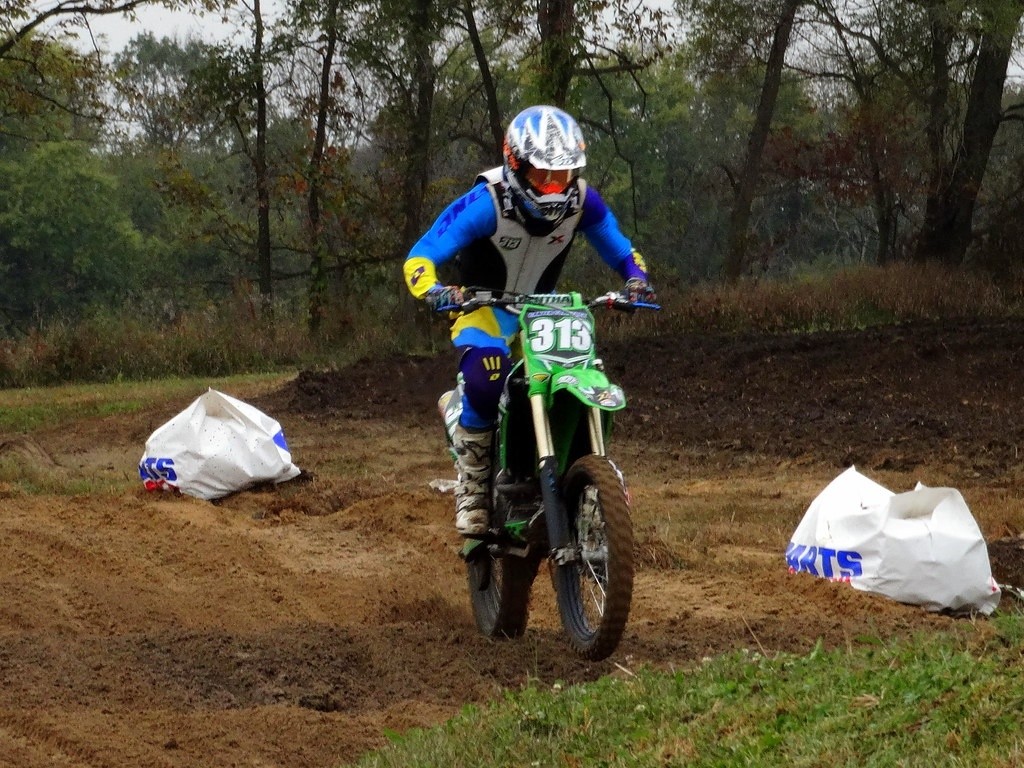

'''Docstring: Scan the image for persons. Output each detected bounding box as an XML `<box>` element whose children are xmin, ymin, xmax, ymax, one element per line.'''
<box><xmin>403</xmin><ymin>104</ymin><xmax>657</xmax><ymax>538</ymax></box>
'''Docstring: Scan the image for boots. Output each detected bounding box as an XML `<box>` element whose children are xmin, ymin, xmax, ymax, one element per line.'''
<box><xmin>584</xmin><ymin>487</ymin><xmax>605</xmax><ymax>533</ymax></box>
<box><xmin>452</xmin><ymin>418</ymin><xmax>493</xmax><ymax>534</ymax></box>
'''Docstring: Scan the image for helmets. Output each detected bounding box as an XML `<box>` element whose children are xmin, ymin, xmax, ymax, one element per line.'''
<box><xmin>503</xmin><ymin>103</ymin><xmax>591</xmax><ymax>239</ymax></box>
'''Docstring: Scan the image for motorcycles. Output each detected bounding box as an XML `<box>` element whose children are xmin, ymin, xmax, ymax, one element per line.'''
<box><xmin>429</xmin><ymin>289</ymin><xmax>662</xmax><ymax>661</ymax></box>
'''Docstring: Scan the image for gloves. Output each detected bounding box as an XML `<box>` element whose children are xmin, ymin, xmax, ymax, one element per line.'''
<box><xmin>624</xmin><ymin>274</ymin><xmax>658</xmax><ymax>305</ymax></box>
<box><xmin>427</xmin><ymin>284</ymin><xmax>464</xmax><ymax>308</ymax></box>
